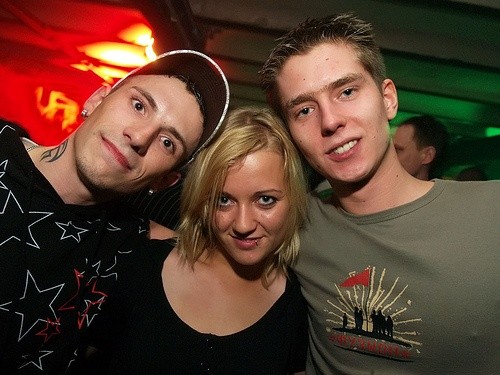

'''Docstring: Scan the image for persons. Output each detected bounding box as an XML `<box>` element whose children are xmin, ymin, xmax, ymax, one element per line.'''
<box><xmin>0</xmin><ymin>9</ymin><xmax>500</xmax><ymax>375</ymax></box>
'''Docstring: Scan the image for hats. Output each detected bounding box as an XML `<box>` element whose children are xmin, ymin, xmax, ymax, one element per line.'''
<box><xmin>117</xmin><ymin>48</ymin><xmax>229</xmax><ymax>170</ymax></box>
